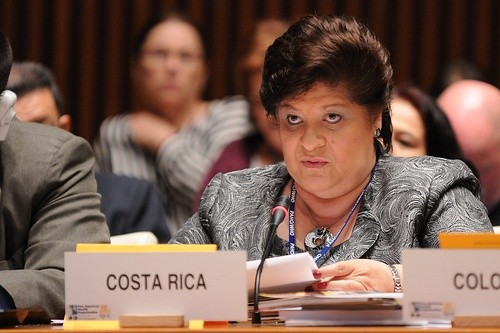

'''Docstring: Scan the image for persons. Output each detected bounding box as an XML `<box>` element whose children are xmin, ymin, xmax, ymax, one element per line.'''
<box><xmin>7</xmin><ymin>60</ymin><xmax>70</xmax><ymax>135</ymax></box>
<box><xmin>167</xmin><ymin>13</ymin><xmax>495</xmax><ymax>293</ymax></box>
<box><xmin>95</xmin><ymin>17</ymin><xmax>254</xmax><ymax>243</ymax></box>
<box><xmin>386</xmin><ymin>82</ymin><xmax>481</xmax><ymax>182</ymax></box>
<box><xmin>0</xmin><ymin>16</ymin><xmax>110</xmax><ymax>319</ymax></box>
<box><xmin>192</xmin><ymin>17</ymin><xmax>285</xmax><ymax>212</ymax></box>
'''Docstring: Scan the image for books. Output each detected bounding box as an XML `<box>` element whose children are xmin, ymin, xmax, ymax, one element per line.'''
<box><xmin>247</xmin><ymin>293</ymin><xmax>454</xmax><ymax>328</ymax></box>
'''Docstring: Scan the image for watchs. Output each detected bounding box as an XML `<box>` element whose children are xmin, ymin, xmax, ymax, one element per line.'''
<box><xmin>387</xmin><ymin>263</ymin><xmax>403</xmax><ymax>293</ymax></box>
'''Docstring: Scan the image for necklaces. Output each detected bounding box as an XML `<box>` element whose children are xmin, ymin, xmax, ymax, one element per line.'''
<box><xmin>303</xmin><ymin>200</ymin><xmax>352</xmax><ymax>250</ymax></box>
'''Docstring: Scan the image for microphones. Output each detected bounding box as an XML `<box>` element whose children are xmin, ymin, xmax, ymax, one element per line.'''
<box><xmin>252</xmin><ymin>194</ymin><xmax>290</xmax><ymax>325</ymax></box>
